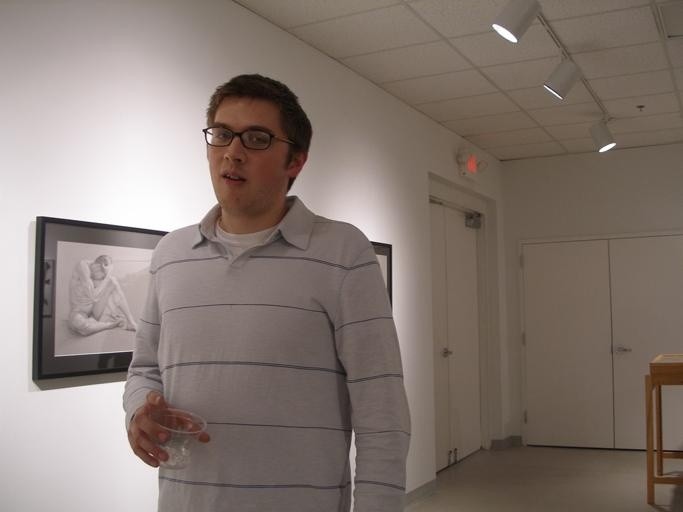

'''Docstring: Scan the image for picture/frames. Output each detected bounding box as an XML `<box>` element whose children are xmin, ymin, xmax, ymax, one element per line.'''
<box><xmin>32</xmin><ymin>215</ymin><xmax>392</xmax><ymax>380</ymax></box>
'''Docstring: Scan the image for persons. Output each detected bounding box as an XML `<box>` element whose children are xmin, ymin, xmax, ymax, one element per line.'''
<box><xmin>120</xmin><ymin>69</ymin><xmax>414</xmax><ymax>512</ymax></box>
<box><xmin>66</xmin><ymin>255</ymin><xmax>140</xmax><ymax>335</ymax></box>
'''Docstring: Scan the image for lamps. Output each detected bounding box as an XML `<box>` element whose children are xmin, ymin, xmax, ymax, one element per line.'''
<box><xmin>492</xmin><ymin>0</ymin><xmax>617</xmax><ymax>154</ymax></box>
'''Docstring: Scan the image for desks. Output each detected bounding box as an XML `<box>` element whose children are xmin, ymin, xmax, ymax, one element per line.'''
<box><xmin>644</xmin><ymin>354</ymin><xmax>683</xmax><ymax>505</ymax></box>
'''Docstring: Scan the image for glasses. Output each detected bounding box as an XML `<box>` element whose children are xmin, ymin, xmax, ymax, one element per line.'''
<box><xmin>202</xmin><ymin>125</ymin><xmax>293</xmax><ymax>150</ymax></box>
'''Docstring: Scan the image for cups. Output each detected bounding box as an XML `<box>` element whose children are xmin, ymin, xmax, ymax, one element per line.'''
<box><xmin>149</xmin><ymin>408</ymin><xmax>206</xmax><ymax>470</ymax></box>
